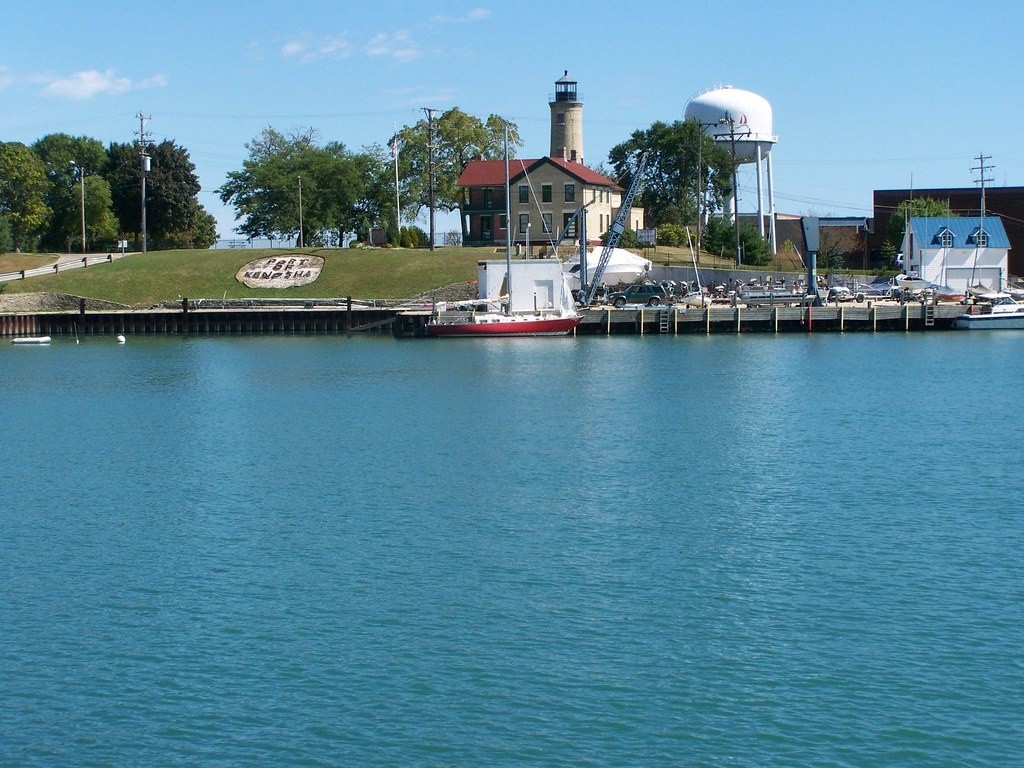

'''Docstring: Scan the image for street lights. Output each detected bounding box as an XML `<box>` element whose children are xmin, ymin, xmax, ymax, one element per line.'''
<box><xmin>69</xmin><ymin>160</ymin><xmax>86</xmax><ymax>254</ymax></box>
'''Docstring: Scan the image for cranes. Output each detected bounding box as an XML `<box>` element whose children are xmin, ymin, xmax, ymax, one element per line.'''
<box><xmin>546</xmin><ymin>151</ymin><xmax>649</xmax><ymax>306</ymax></box>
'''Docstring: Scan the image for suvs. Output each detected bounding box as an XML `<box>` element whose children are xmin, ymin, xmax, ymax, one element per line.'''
<box><xmin>609</xmin><ymin>282</ymin><xmax>668</xmax><ymax>307</ymax></box>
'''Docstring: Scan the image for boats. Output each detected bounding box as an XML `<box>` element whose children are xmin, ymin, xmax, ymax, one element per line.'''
<box><xmin>424</xmin><ymin>121</ymin><xmax>586</xmax><ymax>338</ymax></box>
<box><xmin>895</xmin><ymin>272</ymin><xmax>932</xmax><ymax>292</ymax></box>
<box><xmin>953</xmin><ymin>292</ymin><xmax>1024</xmax><ymax>331</ymax></box>
